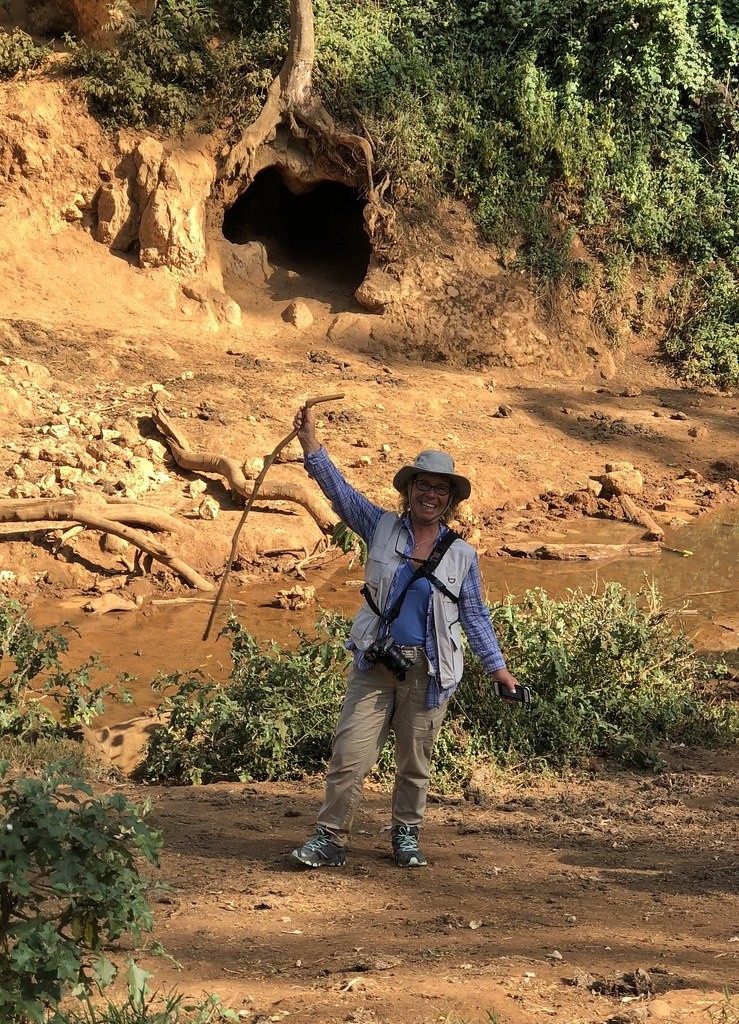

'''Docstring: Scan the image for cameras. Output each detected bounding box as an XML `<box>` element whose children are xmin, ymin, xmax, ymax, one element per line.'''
<box><xmin>364</xmin><ymin>634</ymin><xmax>415</xmax><ymax>682</ymax></box>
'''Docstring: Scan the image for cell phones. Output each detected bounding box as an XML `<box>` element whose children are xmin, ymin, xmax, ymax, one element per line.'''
<box><xmin>493</xmin><ymin>680</ymin><xmax>531</xmax><ymax>703</ymax></box>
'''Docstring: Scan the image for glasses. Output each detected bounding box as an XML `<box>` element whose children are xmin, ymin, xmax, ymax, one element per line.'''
<box><xmin>411</xmin><ymin>479</ymin><xmax>454</xmax><ymax>495</ymax></box>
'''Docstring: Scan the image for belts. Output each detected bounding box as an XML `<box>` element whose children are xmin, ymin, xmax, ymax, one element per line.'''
<box><xmin>395</xmin><ymin>645</ymin><xmax>439</xmax><ymax>677</ymax></box>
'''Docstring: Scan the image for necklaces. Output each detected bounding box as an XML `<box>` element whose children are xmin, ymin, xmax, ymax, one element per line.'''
<box><xmin>414</xmin><ymin>528</ymin><xmax>440</xmax><ymax>549</ymax></box>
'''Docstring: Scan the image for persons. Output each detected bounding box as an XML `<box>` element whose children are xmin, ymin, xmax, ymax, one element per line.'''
<box><xmin>288</xmin><ymin>405</ymin><xmax>520</xmax><ymax>867</ymax></box>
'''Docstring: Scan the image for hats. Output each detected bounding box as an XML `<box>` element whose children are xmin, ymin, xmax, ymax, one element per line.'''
<box><xmin>393</xmin><ymin>450</ymin><xmax>471</xmax><ymax>508</ymax></box>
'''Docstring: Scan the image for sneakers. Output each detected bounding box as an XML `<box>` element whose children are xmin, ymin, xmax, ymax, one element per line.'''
<box><xmin>391</xmin><ymin>825</ymin><xmax>428</xmax><ymax>868</ymax></box>
<box><xmin>290</xmin><ymin>826</ymin><xmax>347</xmax><ymax>867</ymax></box>
